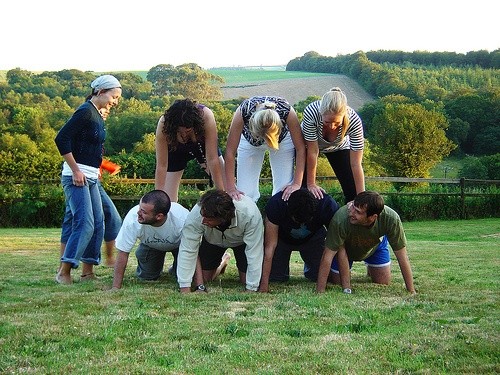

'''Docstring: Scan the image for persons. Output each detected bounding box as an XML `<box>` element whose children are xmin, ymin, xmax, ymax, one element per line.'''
<box><xmin>155</xmin><ymin>99</ymin><xmax>223</xmax><ymax>192</ymax></box>
<box><xmin>302</xmin><ymin>88</ymin><xmax>367</xmax><ymax>199</ymax></box>
<box><xmin>259</xmin><ymin>187</ymin><xmax>352</xmax><ymax>295</ymax></box>
<box><xmin>52</xmin><ymin>75</ymin><xmax>122</xmax><ymax>287</ymax></box>
<box><xmin>177</xmin><ymin>189</ymin><xmax>265</xmax><ymax>294</ymax></box>
<box><xmin>317</xmin><ymin>191</ymin><xmax>416</xmax><ymax>294</ymax></box>
<box><xmin>114</xmin><ymin>190</ymin><xmax>207</xmax><ymax>294</ymax></box>
<box><xmin>224</xmin><ymin>96</ymin><xmax>306</xmax><ymax>201</ymax></box>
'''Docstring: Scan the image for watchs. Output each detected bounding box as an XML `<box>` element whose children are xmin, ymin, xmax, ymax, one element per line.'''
<box><xmin>342</xmin><ymin>288</ymin><xmax>352</xmax><ymax>294</ymax></box>
<box><xmin>193</xmin><ymin>284</ymin><xmax>207</xmax><ymax>292</ymax></box>
